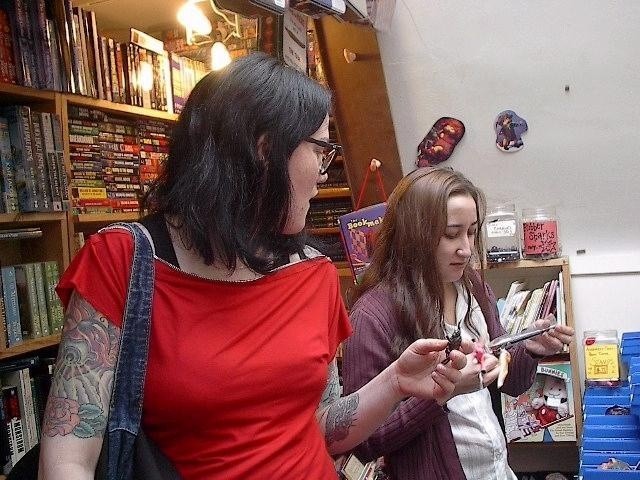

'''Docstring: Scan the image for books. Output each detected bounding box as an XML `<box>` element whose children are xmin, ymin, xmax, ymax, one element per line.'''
<box><xmin>1</xmin><ymin>2</ymin><xmax>360</xmax><ymax>479</ymax></box>
<box><xmin>482</xmin><ymin>270</ymin><xmax>571</xmax><ymax>343</ymax></box>
<box><xmin>336</xmin><ymin>202</ymin><xmax>388</xmax><ymax>288</ymax></box>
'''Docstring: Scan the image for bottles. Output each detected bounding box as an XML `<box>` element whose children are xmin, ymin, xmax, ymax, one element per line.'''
<box><xmin>482</xmin><ymin>204</ymin><xmax>519</xmax><ymax>262</ymax></box>
<box><xmin>582</xmin><ymin>328</ymin><xmax>622</xmax><ymax>388</ymax></box>
<box><xmin>521</xmin><ymin>208</ymin><xmax>561</xmax><ymax>260</ymax></box>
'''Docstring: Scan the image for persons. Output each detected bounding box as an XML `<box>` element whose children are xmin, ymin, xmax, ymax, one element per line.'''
<box><xmin>38</xmin><ymin>53</ymin><xmax>472</xmax><ymax>480</ymax></box>
<box><xmin>337</xmin><ymin>166</ymin><xmax>572</xmax><ymax>479</ymax></box>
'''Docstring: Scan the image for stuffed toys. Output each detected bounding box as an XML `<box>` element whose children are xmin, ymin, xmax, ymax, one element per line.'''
<box><xmin>530</xmin><ymin>374</ymin><xmax>569</xmax><ymax>427</ymax></box>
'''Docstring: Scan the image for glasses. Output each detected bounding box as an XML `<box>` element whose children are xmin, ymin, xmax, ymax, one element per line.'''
<box><xmin>304</xmin><ymin>137</ymin><xmax>341</xmax><ymax>175</ymax></box>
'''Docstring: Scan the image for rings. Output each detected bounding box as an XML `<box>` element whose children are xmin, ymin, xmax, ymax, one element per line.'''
<box><xmin>477</xmin><ymin>367</ymin><xmax>488</xmax><ymax>380</ymax></box>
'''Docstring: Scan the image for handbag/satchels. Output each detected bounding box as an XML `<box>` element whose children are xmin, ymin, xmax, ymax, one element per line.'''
<box><xmin>4</xmin><ymin>424</ymin><xmax>182</xmax><ymax>480</ymax></box>
